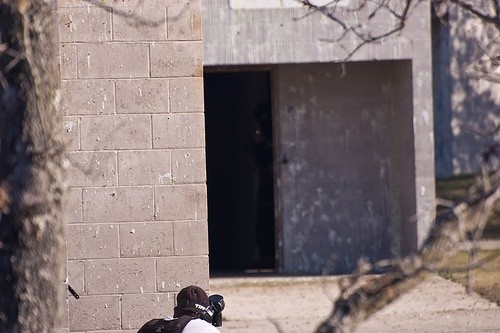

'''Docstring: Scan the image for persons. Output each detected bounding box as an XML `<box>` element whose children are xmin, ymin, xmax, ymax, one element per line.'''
<box><xmin>137</xmin><ymin>286</ymin><xmax>223</xmax><ymax>333</ymax></box>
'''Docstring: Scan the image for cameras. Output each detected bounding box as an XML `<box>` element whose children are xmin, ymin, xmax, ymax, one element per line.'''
<box><xmin>207</xmin><ymin>295</ymin><xmax>225</xmax><ymax>315</ymax></box>
